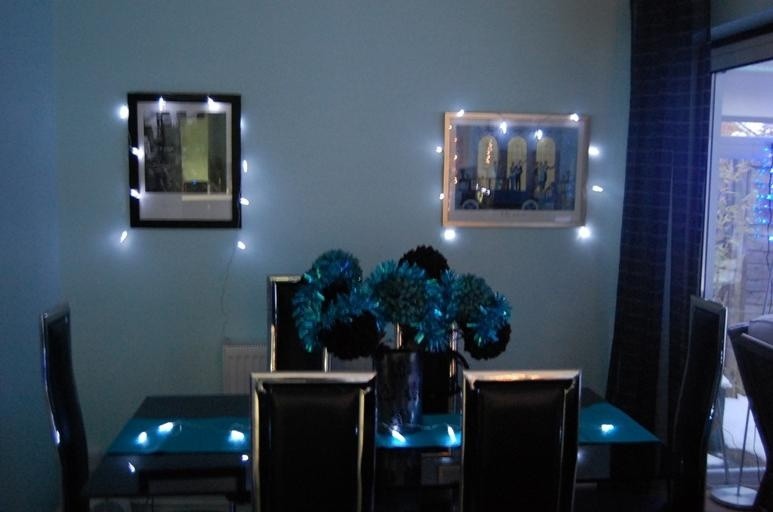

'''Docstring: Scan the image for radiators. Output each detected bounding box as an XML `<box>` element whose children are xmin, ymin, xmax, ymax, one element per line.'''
<box><xmin>220</xmin><ymin>340</ymin><xmax>268</xmax><ymax>395</ymax></box>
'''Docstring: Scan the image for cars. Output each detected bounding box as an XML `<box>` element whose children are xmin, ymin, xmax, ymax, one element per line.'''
<box><xmin>460</xmin><ymin>178</ymin><xmax>538</xmax><ymax>210</ymax></box>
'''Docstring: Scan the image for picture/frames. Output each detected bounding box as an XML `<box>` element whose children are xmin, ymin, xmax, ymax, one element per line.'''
<box><xmin>126</xmin><ymin>91</ymin><xmax>242</xmax><ymax>229</ymax></box>
<box><xmin>443</xmin><ymin>110</ymin><xmax>589</xmax><ymax>228</ymax></box>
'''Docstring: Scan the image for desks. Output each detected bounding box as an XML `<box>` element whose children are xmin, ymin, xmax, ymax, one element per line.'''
<box><xmin>106</xmin><ymin>396</ymin><xmax>662</xmax><ymax>512</ymax></box>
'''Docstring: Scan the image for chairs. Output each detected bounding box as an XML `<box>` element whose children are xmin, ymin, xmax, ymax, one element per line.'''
<box><xmin>270</xmin><ymin>282</ymin><xmax>377</xmax><ymax>418</ymax></box>
<box><xmin>595</xmin><ymin>294</ymin><xmax>729</xmax><ymax>511</ymax></box>
<box><xmin>729</xmin><ymin>323</ymin><xmax>773</xmax><ymax>511</ymax></box>
<box><xmin>38</xmin><ymin>302</ymin><xmax>101</xmax><ymax>511</ymax></box>
<box><xmin>459</xmin><ymin>369</ymin><xmax>582</xmax><ymax>512</ymax></box>
<box><xmin>244</xmin><ymin>369</ymin><xmax>377</xmax><ymax>512</ymax></box>
